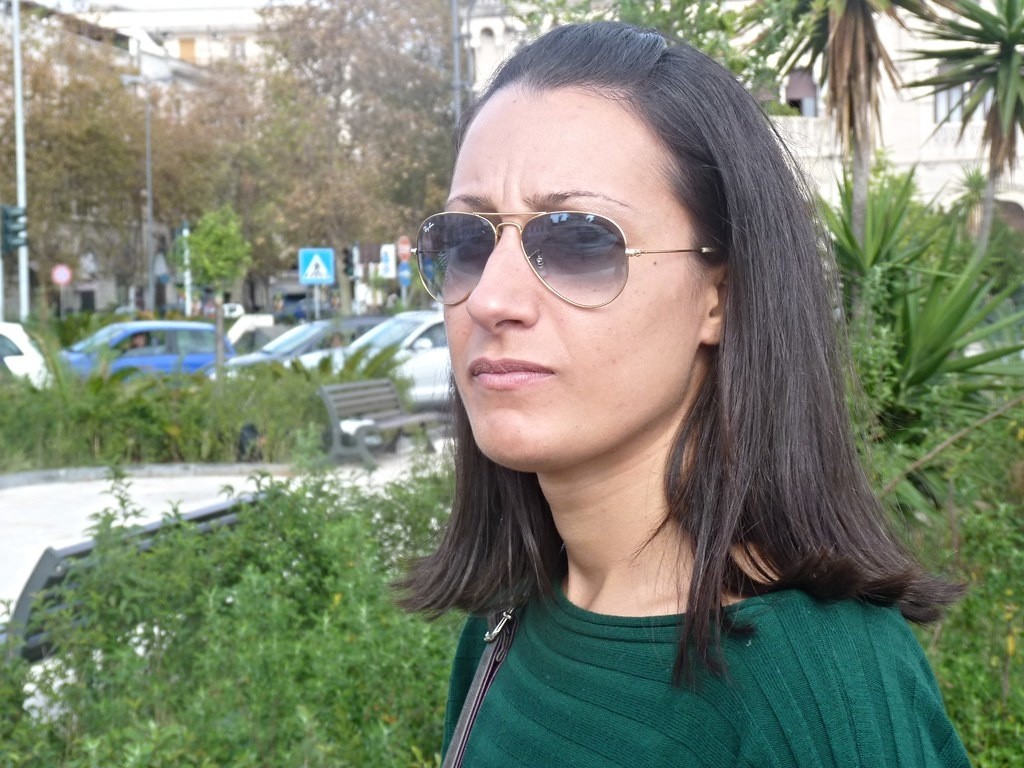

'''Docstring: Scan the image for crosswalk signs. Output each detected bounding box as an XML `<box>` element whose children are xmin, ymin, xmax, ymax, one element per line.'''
<box><xmin>296</xmin><ymin>247</ymin><xmax>337</xmax><ymax>288</ymax></box>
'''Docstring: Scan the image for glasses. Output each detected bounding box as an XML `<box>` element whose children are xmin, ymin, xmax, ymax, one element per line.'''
<box><xmin>411</xmin><ymin>211</ymin><xmax>716</xmax><ymax>310</ymax></box>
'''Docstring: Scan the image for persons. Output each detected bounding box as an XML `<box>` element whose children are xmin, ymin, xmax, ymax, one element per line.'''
<box><xmin>130</xmin><ymin>333</ymin><xmax>149</xmax><ymax>357</ymax></box>
<box><xmin>331</xmin><ymin>331</ymin><xmax>343</xmax><ymax>347</ymax></box>
<box><xmin>385</xmin><ymin>20</ymin><xmax>976</xmax><ymax>768</ymax></box>
<box><xmin>193</xmin><ymin>295</ymin><xmax>216</xmax><ymax>318</ymax></box>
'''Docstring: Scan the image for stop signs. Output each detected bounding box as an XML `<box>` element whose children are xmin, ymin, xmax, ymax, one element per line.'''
<box><xmin>398</xmin><ymin>236</ymin><xmax>411</xmax><ymax>262</ymax></box>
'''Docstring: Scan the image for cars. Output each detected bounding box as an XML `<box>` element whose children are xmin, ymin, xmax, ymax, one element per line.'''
<box><xmin>58</xmin><ymin>320</ymin><xmax>239</xmax><ymax>390</ymax></box>
<box><xmin>212</xmin><ymin>315</ymin><xmax>393</xmax><ymax>370</ymax></box>
<box><xmin>282</xmin><ymin>309</ymin><xmax>457</xmax><ymax>409</ymax></box>
<box><xmin>0</xmin><ymin>320</ymin><xmax>52</xmax><ymax>393</ymax></box>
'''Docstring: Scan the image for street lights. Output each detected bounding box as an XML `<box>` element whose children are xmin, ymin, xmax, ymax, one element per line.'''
<box><xmin>139</xmin><ymin>73</ymin><xmax>177</xmax><ymax>312</ymax></box>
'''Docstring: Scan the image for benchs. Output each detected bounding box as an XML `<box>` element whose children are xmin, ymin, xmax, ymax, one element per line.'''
<box><xmin>309</xmin><ymin>378</ymin><xmax>445</xmax><ymax>470</ymax></box>
<box><xmin>0</xmin><ymin>482</ymin><xmax>291</xmax><ymax>679</ymax></box>
